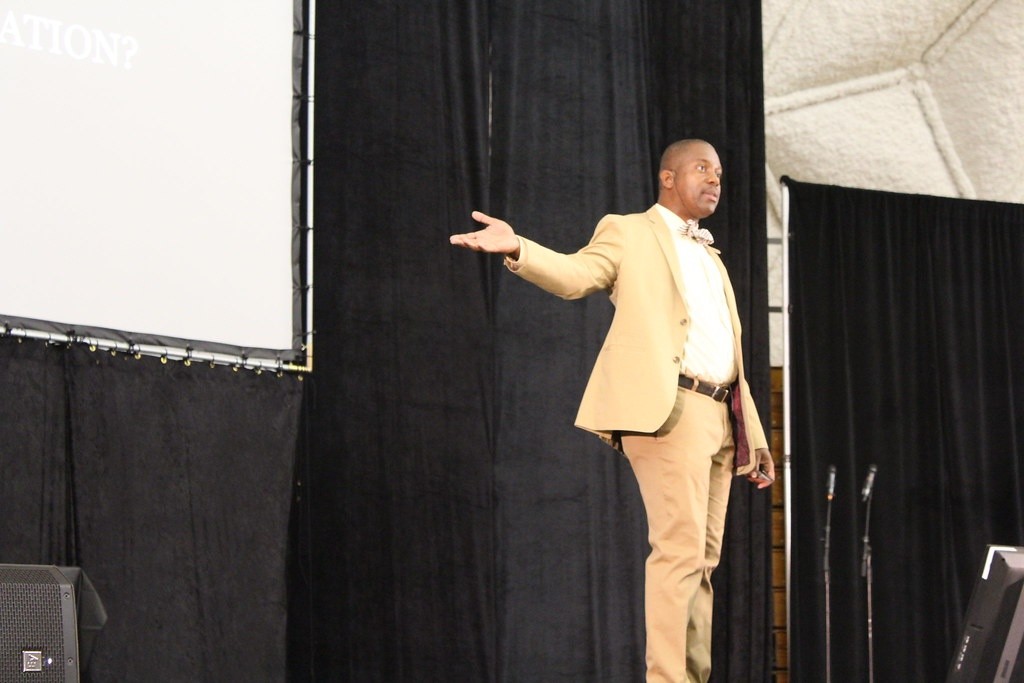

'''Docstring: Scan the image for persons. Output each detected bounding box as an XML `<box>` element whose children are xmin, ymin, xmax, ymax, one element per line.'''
<box><xmin>450</xmin><ymin>139</ymin><xmax>775</xmax><ymax>683</ymax></box>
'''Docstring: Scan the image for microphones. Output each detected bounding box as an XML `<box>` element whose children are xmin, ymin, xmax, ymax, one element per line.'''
<box><xmin>862</xmin><ymin>463</ymin><xmax>878</xmax><ymax>501</ymax></box>
<box><xmin>827</xmin><ymin>466</ymin><xmax>836</xmax><ymax>501</ymax></box>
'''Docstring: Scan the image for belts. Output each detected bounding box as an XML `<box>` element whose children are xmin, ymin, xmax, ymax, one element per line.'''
<box><xmin>677</xmin><ymin>374</ymin><xmax>732</xmax><ymax>404</ymax></box>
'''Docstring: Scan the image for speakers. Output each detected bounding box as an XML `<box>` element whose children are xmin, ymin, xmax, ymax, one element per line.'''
<box><xmin>944</xmin><ymin>544</ymin><xmax>1024</xmax><ymax>683</ymax></box>
<box><xmin>0</xmin><ymin>564</ymin><xmax>80</xmax><ymax>683</ymax></box>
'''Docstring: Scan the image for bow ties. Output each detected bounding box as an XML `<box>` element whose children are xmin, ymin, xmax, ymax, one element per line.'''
<box><xmin>678</xmin><ymin>219</ymin><xmax>715</xmax><ymax>246</ymax></box>
